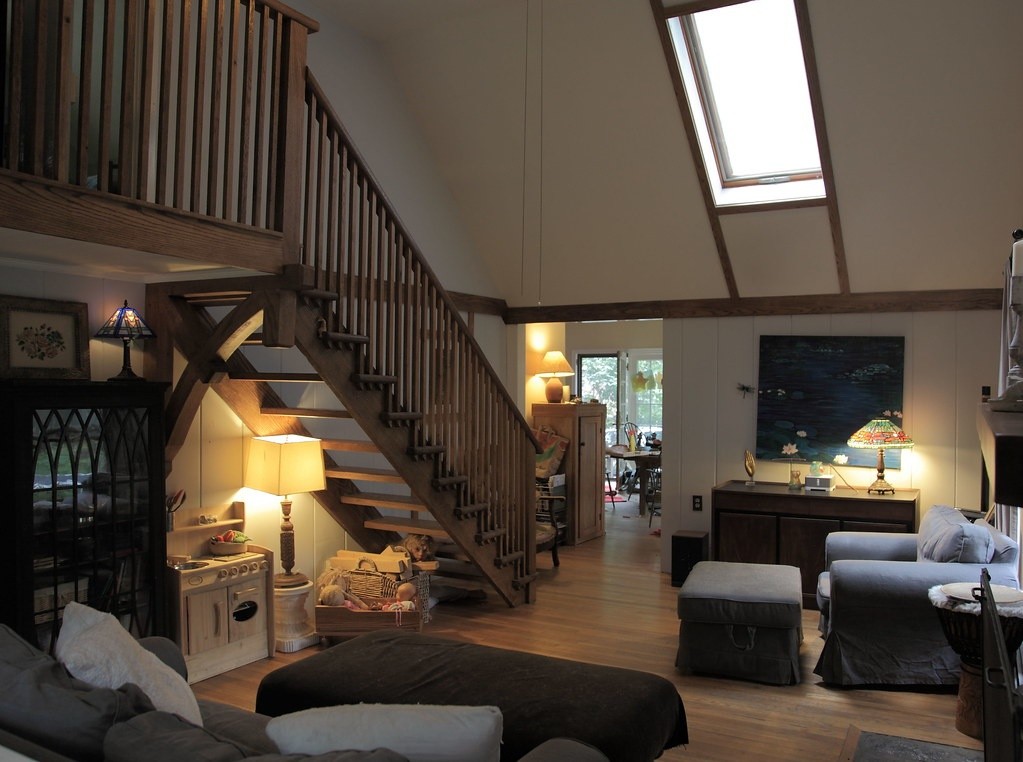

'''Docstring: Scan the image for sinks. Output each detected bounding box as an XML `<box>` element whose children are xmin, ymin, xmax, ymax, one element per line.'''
<box><xmin>173</xmin><ymin>562</ymin><xmax>210</xmax><ymax>570</ymax></box>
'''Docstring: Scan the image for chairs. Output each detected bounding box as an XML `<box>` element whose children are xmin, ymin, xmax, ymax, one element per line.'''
<box><xmin>624</xmin><ymin>421</ymin><xmax>662</xmax><ymax>528</ymax></box>
<box><xmin>535</xmin><ymin>493</ymin><xmax>566</xmax><ymax>567</ymax></box>
<box><xmin>605</xmin><ymin>470</ymin><xmax>617</xmax><ymax>510</ymax></box>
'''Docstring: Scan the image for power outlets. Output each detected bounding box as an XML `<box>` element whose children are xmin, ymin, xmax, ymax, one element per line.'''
<box><xmin>693</xmin><ymin>495</ymin><xmax>702</xmax><ymax>511</ymax></box>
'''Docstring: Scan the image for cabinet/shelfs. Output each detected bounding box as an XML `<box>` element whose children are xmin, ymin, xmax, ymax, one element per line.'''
<box><xmin>531</xmin><ymin>403</ymin><xmax>607</xmax><ymax>546</ymax></box>
<box><xmin>172</xmin><ymin>553</ymin><xmax>275</xmax><ymax>684</ymax></box>
<box><xmin>710</xmin><ymin>480</ymin><xmax>920</xmax><ymax>609</ymax></box>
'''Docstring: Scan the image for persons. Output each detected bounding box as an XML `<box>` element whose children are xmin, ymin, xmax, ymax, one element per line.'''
<box><xmin>355</xmin><ymin>583</ymin><xmax>416</xmax><ymax>610</ymax></box>
<box><xmin>395</xmin><ymin>533</ymin><xmax>438</xmax><ymax>620</ymax></box>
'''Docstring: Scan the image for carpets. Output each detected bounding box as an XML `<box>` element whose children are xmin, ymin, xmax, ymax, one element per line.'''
<box><xmin>605</xmin><ymin>484</ymin><xmax>625</xmax><ymax>502</ymax></box>
<box><xmin>836</xmin><ymin>725</ymin><xmax>984</xmax><ymax>762</ymax></box>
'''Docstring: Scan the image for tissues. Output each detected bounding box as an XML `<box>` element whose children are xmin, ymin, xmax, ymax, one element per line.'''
<box><xmin>804</xmin><ymin>459</ymin><xmax>837</xmax><ymax>492</ymax></box>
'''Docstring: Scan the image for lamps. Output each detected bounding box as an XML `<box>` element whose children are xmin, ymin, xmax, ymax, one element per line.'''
<box><xmin>243</xmin><ymin>433</ymin><xmax>327</xmax><ymax>588</ymax></box>
<box><xmin>847</xmin><ymin>418</ymin><xmax>914</xmax><ymax>496</ymax></box>
<box><xmin>94</xmin><ymin>300</ymin><xmax>157</xmax><ymax>385</ymax></box>
<box><xmin>533</xmin><ymin>349</ymin><xmax>575</xmax><ymax>403</ymax></box>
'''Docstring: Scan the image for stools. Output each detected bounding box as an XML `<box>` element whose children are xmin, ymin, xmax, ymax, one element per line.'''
<box><xmin>253</xmin><ymin>627</ymin><xmax>688</xmax><ymax>762</ymax></box>
<box><xmin>671</xmin><ymin>531</ymin><xmax>710</xmax><ymax>588</ymax></box>
<box><xmin>674</xmin><ymin>560</ymin><xmax>804</xmax><ymax>686</ymax></box>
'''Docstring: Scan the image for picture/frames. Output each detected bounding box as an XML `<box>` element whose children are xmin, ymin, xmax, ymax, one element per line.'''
<box><xmin>0</xmin><ymin>291</ymin><xmax>90</xmax><ymax>384</ymax></box>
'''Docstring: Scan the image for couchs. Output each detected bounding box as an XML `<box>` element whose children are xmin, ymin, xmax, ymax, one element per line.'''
<box><xmin>816</xmin><ymin>505</ymin><xmax>1019</xmax><ymax>690</ymax></box>
<box><xmin>0</xmin><ymin>615</ymin><xmax>529</xmax><ymax>762</ymax></box>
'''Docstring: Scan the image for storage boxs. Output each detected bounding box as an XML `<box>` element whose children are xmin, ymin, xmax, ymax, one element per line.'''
<box><xmin>606</xmin><ymin>448</ymin><xmax>650</xmax><ymax>458</ymax></box>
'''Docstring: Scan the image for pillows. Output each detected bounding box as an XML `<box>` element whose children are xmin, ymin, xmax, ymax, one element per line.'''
<box><xmin>53</xmin><ymin>600</ymin><xmax>203</xmax><ymax>728</ymax></box>
<box><xmin>265</xmin><ymin>705</ymin><xmax>504</xmax><ymax>762</ymax></box>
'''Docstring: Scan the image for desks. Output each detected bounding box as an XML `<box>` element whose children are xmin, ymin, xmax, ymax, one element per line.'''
<box><xmin>272</xmin><ymin>582</ymin><xmax>320</xmax><ymax>653</ymax></box>
<box><xmin>610</xmin><ymin>454</ymin><xmax>661</xmax><ymax>516</ymax></box>
<box><xmin>977</xmin><ymin>404</ymin><xmax>1023</xmax><ymax>507</ymax></box>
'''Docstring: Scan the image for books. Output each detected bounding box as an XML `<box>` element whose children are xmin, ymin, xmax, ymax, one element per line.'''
<box><xmin>33</xmin><ymin>554</ymin><xmax>90</xmax><ymax>625</ymax></box>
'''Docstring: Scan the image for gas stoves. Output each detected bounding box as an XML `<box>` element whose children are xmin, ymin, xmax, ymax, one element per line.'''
<box><xmin>167</xmin><ymin>537</ymin><xmax>271</xmax><ymax>590</ymax></box>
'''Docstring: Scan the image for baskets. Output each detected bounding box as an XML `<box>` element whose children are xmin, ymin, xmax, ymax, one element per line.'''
<box><xmin>397</xmin><ymin>574</ymin><xmax>430</xmax><ymax>623</ymax></box>
<box><xmin>348</xmin><ymin>558</ymin><xmax>397</xmax><ymax>598</ymax></box>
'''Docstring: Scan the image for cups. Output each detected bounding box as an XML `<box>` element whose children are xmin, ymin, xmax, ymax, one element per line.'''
<box><xmin>166</xmin><ymin>512</ymin><xmax>174</xmax><ymax>532</ymax></box>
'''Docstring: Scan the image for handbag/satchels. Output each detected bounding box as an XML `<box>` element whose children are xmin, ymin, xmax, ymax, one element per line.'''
<box><xmin>530</xmin><ymin>424</ymin><xmax>568</xmax><ymax>479</ymax></box>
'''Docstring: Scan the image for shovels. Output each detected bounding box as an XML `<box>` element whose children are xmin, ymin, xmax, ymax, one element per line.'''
<box><xmin>166</xmin><ymin>489</ymin><xmax>187</xmax><ymax>512</ymax></box>
